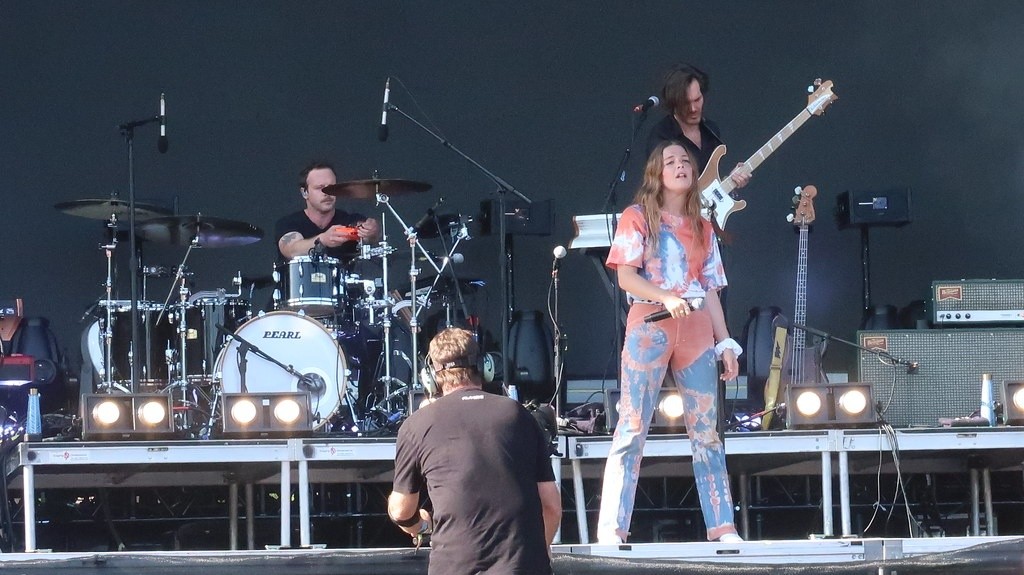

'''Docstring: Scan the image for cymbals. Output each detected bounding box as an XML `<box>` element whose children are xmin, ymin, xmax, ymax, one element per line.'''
<box><xmin>135</xmin><ymin>215</ymin><xmax>265</xmax><ymax>248</ymax></box>
<box><xmin>321</xmin><ymin>179</ymin><xmax>434</xmax><ymax>200</ymax></box>
<box><xmin>53</xmin><ymin>199</ymin><xmax>173</xmax><ymax>222</ymax></box>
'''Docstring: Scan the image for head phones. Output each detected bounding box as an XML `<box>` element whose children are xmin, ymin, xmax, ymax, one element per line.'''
<box><xmin>420</xmin><ymin>351</ymin><xmax>495</xmax><ymax>395</ymax></box>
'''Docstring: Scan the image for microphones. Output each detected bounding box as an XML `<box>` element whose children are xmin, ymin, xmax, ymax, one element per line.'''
<box><xmin>415</xmin><ymin>196</ymin><xmax>445</xmax><ymax>228</ymax></box>
<box><xmin>378</xmin><ymin>77</ymin><xmax>391</xmax><ymax>142</ymax></box>
<box><xmin>643</xmin><ymin>297</ymin><xmax>705</xmax><ymax>323</ymax></box>
<box><xmin>303</xmin><ymin>378</ymin><xmax>324</xmax><ymax>392</ymax></box>
<box><xmin>552</xmin><ymin>246</ymin><xmax>567</xmax><ymax>279</ymax></box>
<box><xmin>906</xmin><ymin>363</ymin><xmax>919</xmax><ymax>375</ymax></box>
<box><xmin>77</xmin><ymin>293</ymin><xmax>107</xmax><ymax>324</ymax></box>
<box><xmin>633</xmin><ymin>95</ymin><xmax>659</xmax><ymax>112</ymax></box>
<box><xmin>158</xmin><ymin>92</ymin><xmax>169</xmax><ymax>153</ymax></box>
<box><xmin>417</xmin><ymin>253</ymin><xmax>464</xmax><ymax>264</ymax></box>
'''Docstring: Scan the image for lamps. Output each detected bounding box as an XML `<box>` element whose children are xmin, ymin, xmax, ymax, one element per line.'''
<box><xmin>737</xmin><ymin>304</ymin><xmax>795</xmax><ymax>414</ymax></box>
<box><xmin>10</xmin><ymin>317</ymin><xmax>69</xmax><ymax>422</ymax></box>
<box><xmin>500</xmin><ymin>309</ymin><xmax>566</xmax><ymax>412</ymax></box>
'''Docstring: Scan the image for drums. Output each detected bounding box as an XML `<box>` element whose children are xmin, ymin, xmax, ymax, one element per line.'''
<box><xmin>344</xmin><ymin>254</ymin><xmax>395</xmax><ymax>316</ymax></box>
<box><xmin>99</xmin><ymin>304</ymin><xmax>168</xmax><ymax>387</ymax></box>
<box><xmin>168</xmin><ymin>301</ymin><xmax>253</xmax><ymax>384</ymax></box>
<box><xmin>213</xmin><ymin>311</ymin><xmax>347</xmax><ymax>430</ymax></box>
<box><xmin>272</xmin><ymin>257</ymin><xmax>347</xmax><ymax>318</ymax></box>
<box><xmin>318</xmin><ymin>317</ymin><xmax>361</xmax><ymax>340</ymax></box>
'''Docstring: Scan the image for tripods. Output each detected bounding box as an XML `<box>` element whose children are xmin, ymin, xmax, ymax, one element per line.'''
<box><xmin>94</xmin><ymin>205</ymin><xmax>222</xmax><ymax>430</ymax></box>
<box><xmin>356</xmin><ymin>184</ymin><xmax>445</xmax><ymax>426</ymax></box>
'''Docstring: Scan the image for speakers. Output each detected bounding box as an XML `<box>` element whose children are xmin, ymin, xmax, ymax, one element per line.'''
<box><xmin>836</xmin><ymin>187</ymin><xmax>912</xmax><ymax>229</ymax></box>
<box><xmin>481</xmin><ymin>199</ymin><xmax>556</xmax><ymax>237</ymax></box>
<box><xmin>930</xmin><ymin>279</ymin><xmax>1024</xmax><ymax>328</ymax></box>
<box><xmin>856</xmin><ymin>328</ymin><xmax>1024</xmax><ymax>428</ymax></box>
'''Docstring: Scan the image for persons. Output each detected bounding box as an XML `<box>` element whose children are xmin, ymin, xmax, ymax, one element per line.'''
<box><xmin>275</xmin><ymin>159</ymin><xmax>385</xmax><ymax>427</ymax></box>
<box><xmin>388</xmin><ymin>327</ymin><xmax>563</xmax><ymax>575</ymax></box>
<box><xmin>597</xmin><ymin>62</ymin><xmax>752</xmax><ymax>546</ymax></box>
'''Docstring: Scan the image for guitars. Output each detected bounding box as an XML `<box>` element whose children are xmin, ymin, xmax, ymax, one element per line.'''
<box><xmin>697</xmin><ymin>77</ymin><xmax>839</xmax><ymax>241</ymax></box>
<box><xmin>764</xmin><ymin>184</ymin><xmax>829</xmax><ymax>421</ymax></box>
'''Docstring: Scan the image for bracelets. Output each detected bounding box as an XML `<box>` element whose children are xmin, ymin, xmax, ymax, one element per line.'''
<box><xmin>713</xmin><ymin>337</ymin><xmax>743</xmax><ymax>360</ymax></box>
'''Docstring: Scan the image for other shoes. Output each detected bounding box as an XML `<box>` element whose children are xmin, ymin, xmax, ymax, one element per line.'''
<box><xmin>717</xmin><ymin>533</ymin><xmax>743</xmax><ymax>543</ymax></box>
<box><xmin>598</xmin><ymin>535</ymin><xmax>624</xmax><ymax>544</ymax></box>
